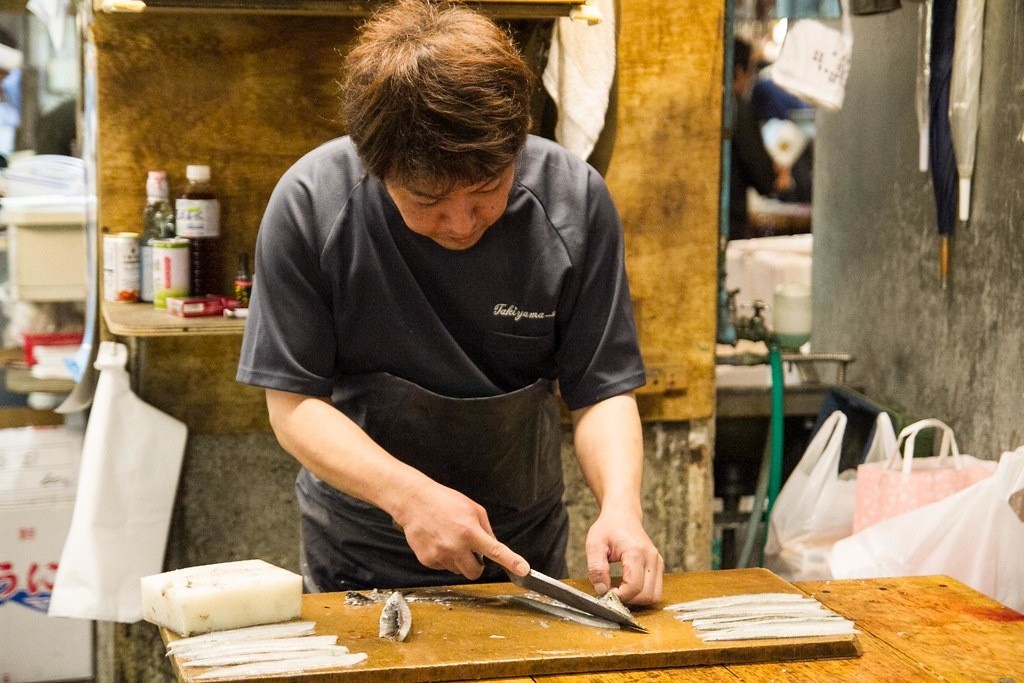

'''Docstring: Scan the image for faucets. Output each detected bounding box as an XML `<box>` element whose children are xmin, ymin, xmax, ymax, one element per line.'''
<box><xmin>736</xmin><ymin>300</ymin><xmax>782</xmax><ymax>350</ymax></box>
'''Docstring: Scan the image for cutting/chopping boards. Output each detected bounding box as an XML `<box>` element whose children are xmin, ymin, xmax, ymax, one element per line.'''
<box><xmin>158</xmin><ymin>567</ymin><xmax>861</xmax><ymax>682</ymax></box>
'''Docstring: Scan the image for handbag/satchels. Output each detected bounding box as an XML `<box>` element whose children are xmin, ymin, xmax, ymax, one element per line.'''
<box><xmin>763</xmin><ymin>410</ymin><xmax>1024</xmax><ymax>614</ymax></box>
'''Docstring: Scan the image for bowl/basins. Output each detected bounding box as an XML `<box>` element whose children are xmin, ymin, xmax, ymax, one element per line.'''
<box><xmin>21</xmin><ymin>332</ymin><xmax>82</xmax><ymax>366</ymax></box>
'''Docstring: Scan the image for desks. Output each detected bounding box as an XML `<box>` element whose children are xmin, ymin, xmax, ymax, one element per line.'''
<box><xmin>157</xmin><ymin>564</ymin><xmax>1024</xmax><ymax>683</ymax></box>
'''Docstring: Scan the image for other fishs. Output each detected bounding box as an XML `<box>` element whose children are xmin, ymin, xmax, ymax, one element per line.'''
<box><xmin>379</xmin><ymin>590</ymin><xmax>412</xmax><ymax>643</ymax></box>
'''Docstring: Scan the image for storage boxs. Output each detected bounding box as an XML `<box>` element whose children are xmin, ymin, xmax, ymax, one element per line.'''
<box><xmin>0</xmin><ymin>206</ymin><xmax>88</xmax><ymax>302</ymax></box>
<box><xmin>166</xmin><ymin>295</ymin><xmax>225</xmax><ymax>318</ymax></box>
<box><xmin>811</xmin><ymin>383</ymin><xmax>936</xmax><ymax>472</ymax></box>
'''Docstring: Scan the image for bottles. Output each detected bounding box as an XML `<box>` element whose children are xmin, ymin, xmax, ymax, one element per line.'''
<box><xmin>176</xmin><ymin>165</ymin><xmax>221</xmax><ymax>298</ymax></box>
<box><xmin>737</xmin><ymin>497</ymin><xmax>768</xmax><ymax>570</ymax></box>
<box><xmin>712</xmin><ymin>497</ymin><xmax>724</xmax><ymax>571</ymax></box>
<box><xmin>141</xmin><ymin>171</ymin><xmax>175</xmax><ymax>301</ymax></box>
<box><xmin>234</xmin><ymin>252</ymin><xmax>253</xmax><ymax>309</ymax></box>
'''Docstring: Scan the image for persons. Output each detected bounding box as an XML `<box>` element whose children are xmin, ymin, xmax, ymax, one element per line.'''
<box><xmin>730</xmin><ymin>35</ymin><xmax>817</xmax><ymax>244</ymax></box>
<box><xmin>233</xmin><ymin>0</ymin><xmax>665</xmax><ymax>607</ymax></box>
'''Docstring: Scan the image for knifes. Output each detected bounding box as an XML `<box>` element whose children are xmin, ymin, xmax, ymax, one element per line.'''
<box><xmin>475</xmin><ymin>552</ymin><xmax>646</xmax><ymax>631</ymax></box>
